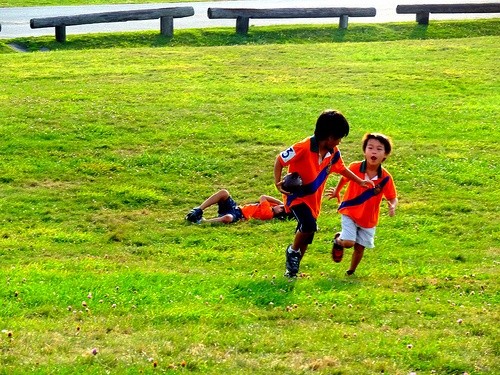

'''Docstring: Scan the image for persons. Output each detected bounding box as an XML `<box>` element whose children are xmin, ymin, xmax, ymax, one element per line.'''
<box><xmin>274</xmin><ymin>110</ymin><xmax>375</xmax><ymax>277</ymax></box>
<box><xmin>185</xmin><ymin>189</ymin><xmax>296</xmax><ymax>222</ymax></box>
<box><xmin>324</xmin><ymin>133</ymin><xmax>398</xmax><ymax>277</ymax></box>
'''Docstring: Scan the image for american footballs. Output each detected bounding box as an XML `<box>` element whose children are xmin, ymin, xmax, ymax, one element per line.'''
<box><xmin>282</xmin><ymin>172</ymin><xmax>302</xmax><ymax>191</ymax></box>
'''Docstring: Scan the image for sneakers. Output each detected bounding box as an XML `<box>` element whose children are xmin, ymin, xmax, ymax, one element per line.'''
<box><xmin>332</xmin><ymin>232</ymin><xmax>344</xmax><ymax>263</ymax></box>
<box><xmin>284</xmin><ymin>244</ymin><xmax>302</xmax><ymax>273</ymax></box>
<box><xmin>284</xmin><ymin>269</ymin><xmax>299</xmax><ymax>279</ymax></box>
<box><xmin>185</xmin><ymin>206</ymin><xmax>204</xmax><ymax>224</ymax></box>
